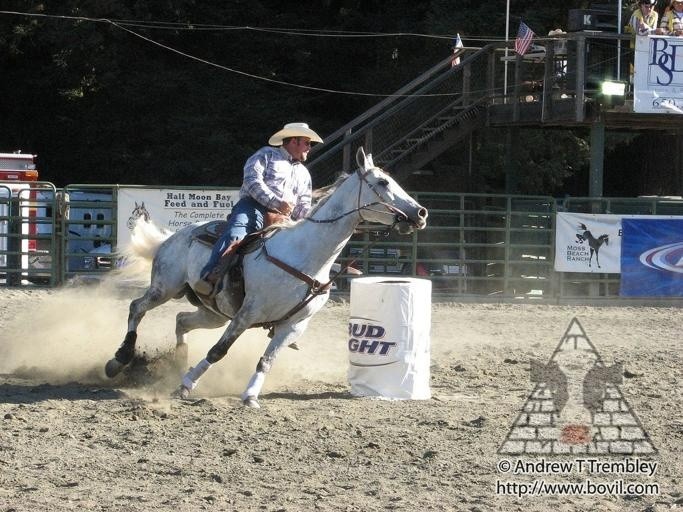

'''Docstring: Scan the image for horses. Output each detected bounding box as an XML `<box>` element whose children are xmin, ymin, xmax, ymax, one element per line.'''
<box><xmin>576</xmin><ymin>222</ymin><xmax>609</xmax><ymax>269</ymax></box>
<box><xmin>104</xmin><ymin>146</ymin><xmax>429</xmax><ymax>408</ymax></box>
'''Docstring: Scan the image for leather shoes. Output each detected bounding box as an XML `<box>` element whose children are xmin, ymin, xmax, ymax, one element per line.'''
<box><xmin>269</xmin><ymin>328</ymin><xmax>301</xmax><ymax>351</ymax></box>
<box><xmin>194</xmin><ymin>278</ymin><xmax>212</xmax><ymax>297</ymax></box>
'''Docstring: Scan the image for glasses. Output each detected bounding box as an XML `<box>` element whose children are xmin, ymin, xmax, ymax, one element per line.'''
<box><xmin>296</xmin><ymin>140</ymin><xmax>311</xmax><ymax>148</ymax></box>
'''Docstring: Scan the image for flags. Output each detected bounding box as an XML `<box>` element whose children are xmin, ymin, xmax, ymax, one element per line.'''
<box><xmin>452</xmin><ymin>33</ymin><xmax>463</xmax><ymax>68</ymax></box>
<box><xmin>514</xmin><ymin>21</ymin><xmax>533</xmax><ymax>55</ymax></box>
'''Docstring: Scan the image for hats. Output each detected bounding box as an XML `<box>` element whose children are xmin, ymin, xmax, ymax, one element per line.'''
<box><xmin>268</xmin><ymin>123</ymin><xmax>325</xmax><ymax>147</ymax></box>
<box><xmin>638</xmin><ymin>0</ymin><xmax>658</xmax><ymax>7</ymax></box>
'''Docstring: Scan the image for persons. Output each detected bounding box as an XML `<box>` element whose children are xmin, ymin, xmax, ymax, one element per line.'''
<box><xmin>193</xmin><ymin>121</ymin><xmax>325</xmax><ymax>350</ymax></box>
<box><xmin>660</xmin><ymin>0</ymin><xmax>683</xmax><ymax>36</ymax></box>
<box><xmin>628</xmin><ymin>1</ymin><xmax>662</xmax><ymax>95</ymax></box>
